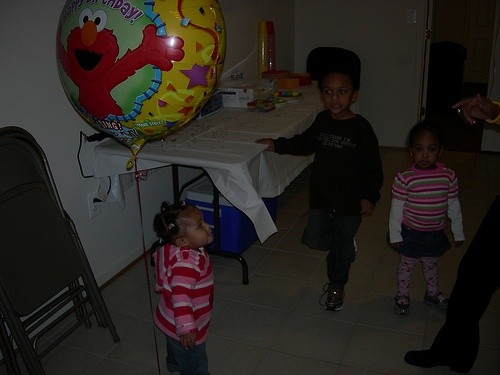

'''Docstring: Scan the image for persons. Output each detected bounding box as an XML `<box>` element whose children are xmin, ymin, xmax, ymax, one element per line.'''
<box><xmin>254</xmin><ymin>62</ymin><xmax>383</xmax><ymax>312</ymax></box>
<box><xmin>385</xmin><ymin>118</ymin><xmax>466</xmax><ymax>315</ymax></box>
<box><xmin>151</xmin><ymin>201</ymin><xmax>214</xmax><ymax>375</ymax></box>
<box><xmin>404</xmin><ymin>93</ymin><xmax>500</xmax><ymax>373</ymax></box>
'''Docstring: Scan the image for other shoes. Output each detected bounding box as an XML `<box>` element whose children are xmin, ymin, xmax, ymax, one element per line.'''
<box><xmin>404</xmin><ymin>344</ymin><xmax>477</xmax><ymax>373</ymax></box>
<box><xmin>424</xmin><ymin>290</ymin><xmax>448</xmax><ymax>309</ymax></box>
<box><xmin>322</xmin><ymin>281</ymin><xmax>345</xmax><ymax>311</ymax></box>
<box><xmin>395</xmin><ymin>295</ymin><xmax>412</xmax><ymax>314</ymax></box>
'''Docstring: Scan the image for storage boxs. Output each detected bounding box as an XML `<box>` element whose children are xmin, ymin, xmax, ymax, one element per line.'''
<box><xmin>184</xmin><ymin>175</ymin><xmax>280</xmax><ymax>255</ymax></box>
<box><xmin>219</xmin><ymin>86</ymin><xmax>255</xmax><ymax>107</ymax></box>
<box><xmin>195</xmin><ymin>89</ymin><xmax>223</xmax><ymax>120</ymax></box>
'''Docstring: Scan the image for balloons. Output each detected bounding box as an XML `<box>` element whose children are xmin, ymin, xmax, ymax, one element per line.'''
<box><xmin>56</xmin><ymin>0</ymin><xmax>227</xmax><ymax>168</ymax></box>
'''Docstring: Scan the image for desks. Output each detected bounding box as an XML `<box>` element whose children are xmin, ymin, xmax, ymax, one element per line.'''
<box><xmin>93</xmin><ymin>80</ymin><xmax>325</xmax><ymax>284</ymax></box>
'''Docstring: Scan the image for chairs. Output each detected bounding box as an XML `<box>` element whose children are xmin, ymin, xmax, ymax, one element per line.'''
<box><xmin>0</xmin><ymin>126</ymin><xmax>119</xmax><ymax>375</ymax></box>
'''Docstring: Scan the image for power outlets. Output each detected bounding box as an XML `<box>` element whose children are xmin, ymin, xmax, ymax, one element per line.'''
<box><xmin>88</xmin><ymin>192</ymin><xmax>101</xmax><ymax>219</ymax></box>
